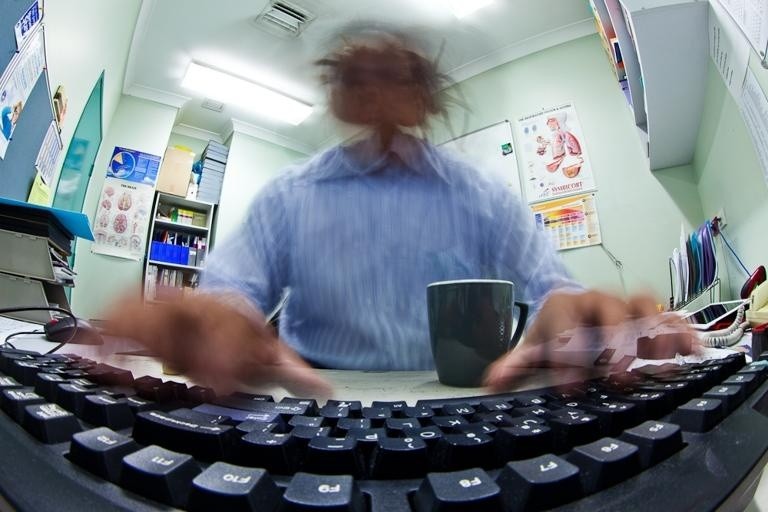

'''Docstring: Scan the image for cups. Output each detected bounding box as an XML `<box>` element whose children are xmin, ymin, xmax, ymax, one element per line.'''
<box><xmin>426</xmin><ymin>278</ymin><xmax>529</xmax><ymax>388</ymax></box>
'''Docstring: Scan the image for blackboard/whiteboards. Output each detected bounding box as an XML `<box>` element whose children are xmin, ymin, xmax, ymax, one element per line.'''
<box><xmin>435</xmin><ymin>119</ymin><xmax>523</xmax><ymax>205</ymax></box>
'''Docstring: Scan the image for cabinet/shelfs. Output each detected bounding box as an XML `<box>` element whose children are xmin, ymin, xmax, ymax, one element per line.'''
<box><xmin>142</xmin><ymin>191</ymin><xmax>216</xmax><ymax>306</ymax></box>
<box><xmin>586</xmin><ymin>0</ymin><xmax>710</xmax><ymax>171</ymax></box>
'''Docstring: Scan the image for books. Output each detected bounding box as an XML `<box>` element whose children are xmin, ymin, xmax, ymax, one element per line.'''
<box><xmin>195</xmin><ymin>139</ymin><xmax>230</xmax><ymax>207</ymax></box>
<box><xmin>143</xmin><ymin>265</ymin><xmax>184</xmax><ymax>302</ymax></box>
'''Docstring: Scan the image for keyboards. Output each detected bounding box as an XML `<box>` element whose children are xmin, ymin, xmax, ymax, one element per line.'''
<box><xmin>0</xmin><ymin>349</ymin><xmax>768</xmax><ymax>512</ymax></box>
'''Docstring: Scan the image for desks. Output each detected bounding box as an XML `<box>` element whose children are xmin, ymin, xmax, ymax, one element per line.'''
<box><xmin>0</xmin><ymin>315</ymin><xmax>767</xmax><ymax>512</ymax></box>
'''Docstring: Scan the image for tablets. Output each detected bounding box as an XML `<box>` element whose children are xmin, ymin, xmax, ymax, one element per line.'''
<box><xmin>680</xmin><ymin>296</ymin><xmax>752</xmax><ymax>330</ymax></box>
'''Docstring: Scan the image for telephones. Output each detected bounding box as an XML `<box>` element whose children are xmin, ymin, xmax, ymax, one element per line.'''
<box><xmin>745</xmin><ymin>280</ymin><xmax>768</xmax><ymax>323</ymax></box>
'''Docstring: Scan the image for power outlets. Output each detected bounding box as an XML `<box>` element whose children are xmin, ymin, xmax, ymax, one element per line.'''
<box><xmin>715</xmin><ymin>207</ymin><xmax>726</xmax><ymax>231</ymax></box>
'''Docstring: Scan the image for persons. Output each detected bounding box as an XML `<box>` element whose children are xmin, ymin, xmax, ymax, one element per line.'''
<box><xmin>76</xmin><ymin>11</ymin><xmax>710</xmax><ymax>402</ymax></box>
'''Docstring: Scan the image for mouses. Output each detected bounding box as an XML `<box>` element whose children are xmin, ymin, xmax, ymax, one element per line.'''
<box><xmin>44</xmin><ymin>319</ymin><xmax>102</xmax><ymax>345</ymax></box>
